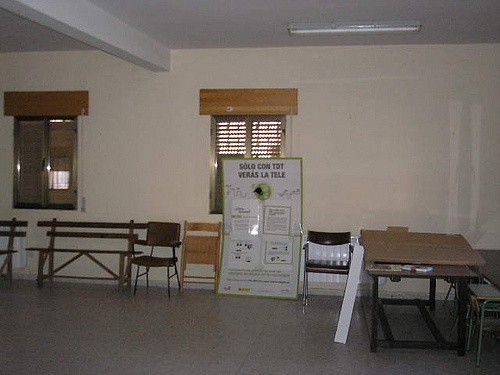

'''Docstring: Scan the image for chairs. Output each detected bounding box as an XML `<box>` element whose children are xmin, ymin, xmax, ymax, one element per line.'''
<box><xmin>181</xmin><ymin>219</ymin><xmax>222</xmax><ymax>292</ymax></box>
<box><xmin>441</xmin><ymin>263</ymin><xmax>500</xmax><ymax>368</ymax></box>
<box><xmin>130</xmin><ymin>222</ymin><xmax>182</xmax><ymax>297</ymax></box>
<box><xmin>301</xmin><ymin>230</ymin><xmax>354</xmax><ymax>307</ymax></box>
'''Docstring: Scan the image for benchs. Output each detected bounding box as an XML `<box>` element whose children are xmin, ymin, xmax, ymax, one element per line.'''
<box><xmin>24</xmin><ymin>217</ymin><xmax>147</xmax><ymax>291</ymax></box>
<box><xmin>0</xmin><ymin>218</ymin><xmax>28</xmax><ymax>281</ymax></box>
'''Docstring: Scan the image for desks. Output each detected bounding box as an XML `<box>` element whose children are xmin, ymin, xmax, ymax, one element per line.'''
<box><xmin>360</xmin><ymin>230</ymin><xmax>500</xmax><ymax>356</ymax></box>
